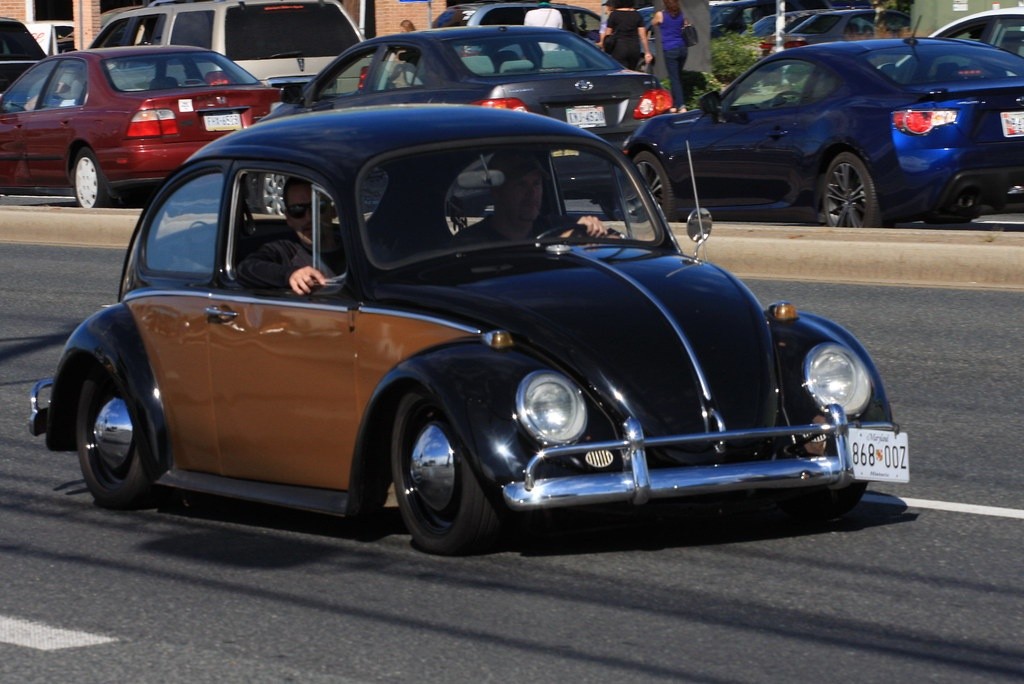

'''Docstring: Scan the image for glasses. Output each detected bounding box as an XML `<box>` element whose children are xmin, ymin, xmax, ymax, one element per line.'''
<box><xmin>286</xmin><ymin>199</ymin><xmax>327</xmax><ymax>219</ymax></box>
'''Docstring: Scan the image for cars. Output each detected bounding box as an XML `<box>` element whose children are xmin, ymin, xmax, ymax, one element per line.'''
<box><xmin>0</xmin><ymin>18</ymin><xmax>48</xmax><ymax>96</ymax></box>
<box><xmin>927</xmin><ymin>7</ymin><xmax>1024</xmax><ymax>57</ymax></box>
<box><xmin>258</xmin><ymin>24</ymin><xmax>674</xmax><ymax>221</ymax></box>
<box><xmin>621</xmin><ymin>37</ymin><xmax>1024</xmax><ymax>230</ymax></box>
<box><xmin>634</xmin><ymin>0</ymin><xmax>873</xmax><ymax>69</ymax></box>
<box><xmin>740</xmin><ymin>7</ymin><xmax>911</xmax><ymax>57</ymax></box>
<box><xmin>431</xmin><ymin>2</ymin><xmax>604</xmax><ymax>45</ymax></box>
<box><xmin>27</xmin><ymin>104</ymin><xmax>912</xmax><ymax>557</ymax></box>
<box><xmin>0</xmin><ymin>44</ymin><xmax>283</xmax><ymax>207</ymax></box>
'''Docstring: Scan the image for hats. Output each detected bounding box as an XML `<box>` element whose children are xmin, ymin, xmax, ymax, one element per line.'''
<box><xmin>601</xmin><ymin>0</ymin><xmax>611</xmax><ymax>6</ymax></box>
<box><xmin>488</xmin><ymin>152</ymin><xmax>550</xmax><ymax>182</ymax></box>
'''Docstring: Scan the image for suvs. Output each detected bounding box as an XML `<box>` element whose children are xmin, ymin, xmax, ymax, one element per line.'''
<box><xmin>88</xmin><ymin>0</ymin><xmax>364</xmax><ymax>103</ymax></box>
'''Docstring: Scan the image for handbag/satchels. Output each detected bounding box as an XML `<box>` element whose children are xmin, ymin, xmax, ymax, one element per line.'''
<box><xmin>603</xmin><ymin>34</ymin><xmax>616</xmax><ymax>53</ymax></box>
<box><xmin>682</xmin><ymin>25</ymin><xmax>698</xmax><ymax>46</ymax></box>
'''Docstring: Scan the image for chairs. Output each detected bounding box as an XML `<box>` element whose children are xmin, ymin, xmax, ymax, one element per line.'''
<box><xmin>149</xmin><ymin>76</ymin><xmax>178</xmax><ymax>91</ymax></box>
<box><xmin>460</xmin><ymin>51</ymin><xmax>579</xmax><ymax>74</ymax></box>
<box><xmin>69</xmin><ymin>80</ymin><xmax>87</xmax><ymax>97</ymax></box>
<box><xmin>878</xmin><ymin>61</ymin><xmax>963</xmax><ymax>85</ymax></box>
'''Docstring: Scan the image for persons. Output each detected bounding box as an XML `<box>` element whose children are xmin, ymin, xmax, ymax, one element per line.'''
<box><xmin>235</xmin><ymin>176</ymin><xmax>345</xmax><ymax>296</ymax></box>
<box><xmin>400</xmin><ymin>20</ymin><xmax>415</xmax><ymax>32</ymax></box>
<box><xmin>450</xmin><ymin>151</ymin><xmax>608</xmax><ymax>251</ymax></box>
<box><xmin>594</xmin><ymin>0</ymin><xmax>653</xmax><ymax>71</ymax></box>
<box><xmin>650</xmin><ymin>0</ymin><xmax>690</xmax><ymax>114</ymax></box>
